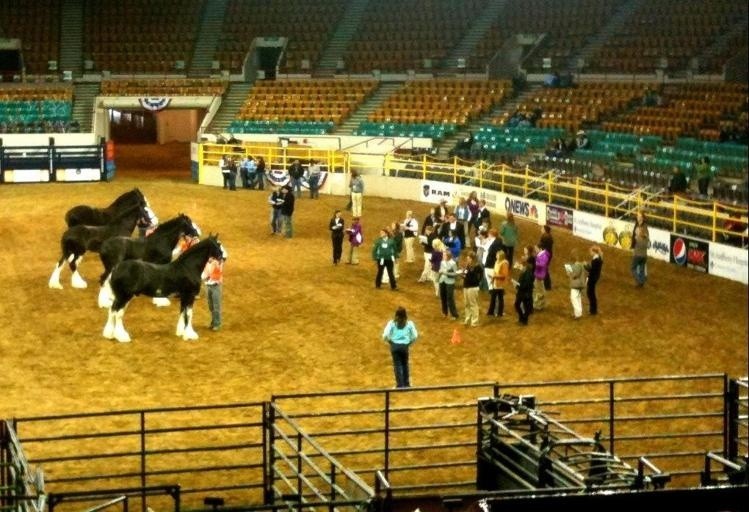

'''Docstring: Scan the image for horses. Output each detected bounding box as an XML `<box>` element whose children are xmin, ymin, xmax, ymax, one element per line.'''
<box><xmin>97</xmin><ymin>211</ymin><xmax>203</xmax><ymax>309</ymax></box>
<box><xmin>101</xmin><ymin>231</ymin><xmax>228</xmax><ymax>343</ymax></box>
<box><xmin>65</xmin><ymin>186</ymin><xmax>151</xmax><ymax>228</ymax></box>
<box><xmin>47</xmin><ymin>200</ymin><xmax>159</xmax><ymax>290</ymax></box>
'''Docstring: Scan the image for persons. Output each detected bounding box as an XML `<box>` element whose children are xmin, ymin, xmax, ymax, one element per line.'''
<box><xmin>345</xmin><ymin>217</ymin><xmax>363</xmax><ymax>265</ymax></box>
<box><xmin>307</xmin><ymin>159</ymin><xmax>320</xmax><ymax>198</ymax></box>
<box><xmin>328</xmin><ymin>209</ymin><xmax>344</xmax><ymax>265</ymax></box>
<box><xmin>178</xmin><ymin>236</ymin><xmax>201</xmax><ymax>299</ymax></box>
<box><xmin>667</xmin><ymin>155</ymin><xmax>748</xmax><ymax>206</ymax></box>
<box><xmin>348</xmin><ymin>168</ymin><xmax>364</xmax><ymax>219</ymax></box>
<box><xmin>267</xmin><ymin>184</ymin><xmax>281</xmax><ymax>236</ymax></box>
<box><xmin>546</xmin><ymin>126</ymin><xmax>590</xmax><ymax>157</ymax></box>
<box><xmin>460</xmin><ymin>130</ymin><xmax>473</xmax><ymax>149</ymax></box>
<box><xmin>345</xmin><ymin>169</ymin><xmax>356</xmax><ymax>211</ymax></box>
<box><xmin>278</xmin><ymin>185</ymin><xmax>294</xmax><ymax>238</ymax></box>
<box><xmin>653</xmin><ymin>87</ymin><xmax>670</xmax><ymax>107</ymax></box>
<box><xmin>629</xmin><ymin>210</ymin><xmax>650</xmax><ymax>279</ymax></box>
<box><xmin>562</xmin><ymin>248</ymin><xmax>585</xmax><ymax>319</ymax></box>
<box><xmin>542</xmin><ymin>70</ymin><xmax>577</xmax><ymax>88</ymax></box>
<box><xmin>505</xmin><ymin>104</ymin><xmax>544</xmax><ymax>127</ymax></box>
<box><xmin>584</xmin><ymin>244</ymin><xmax>604</xmax><ymax>316</ymax></box>
<box><xmin>214</xmin><ymin>130</ymin><xmax>265</xmax><ymax>191</ymax></box>
<box><xmin>200</xmin><ymin>256</ymin><xmax>225</xmax><ymax>332</ymax></box>
<box><xmin>369</xmin><ymin>190</ymin><xmax>555</xmax><ymax>327</ymax></box>
<box><xmin>380</xmin><ymin>305</ymin><xmax>419</xmax><ymax>390</ymax></box>
<box><xmin>642</xmin><ymin>87</ymin><xmax>655</xmax><ymax>107</ymax></box>
<box><xmin>631</xmin><ymin>224</ymin><xmax>648</xmax><ymax>287</ymax></box>
<box><xmin>0</xmin><ymin>119</ymin><xmax>81</xmax><ymax>134</ymax></box>
<box><xmin>288</xmin><ymin>158</ymin><xmax>305</xmax><ymax>198</ymax></box>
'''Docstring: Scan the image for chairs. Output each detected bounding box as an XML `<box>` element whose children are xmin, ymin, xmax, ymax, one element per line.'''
<box><xmin>213</xmin><ymin>1</ymin><xmax>514</xmax><ymax>145</ymax></box>
<box><xmin>453</xmin><ymin>0</ymin><xmax>749</xmax><ymax>253</ymax></box>
<box><xmin>0</xmin><ymin>1</ymin><xmax>208</xmax><ymax>133</ymax></box>
